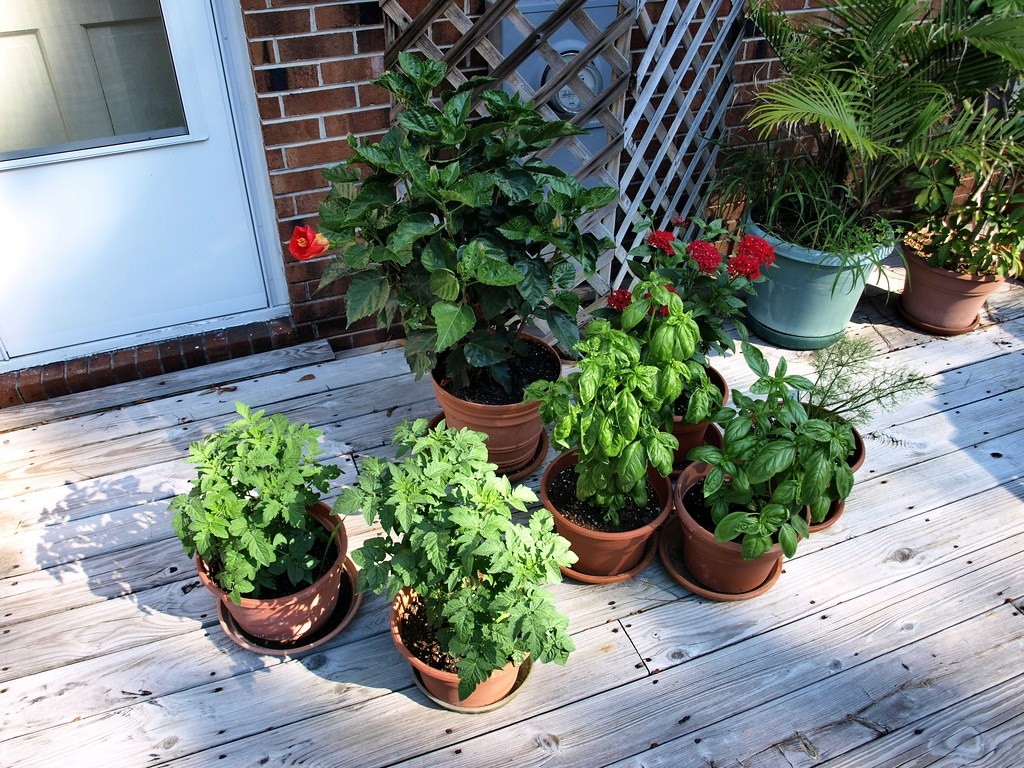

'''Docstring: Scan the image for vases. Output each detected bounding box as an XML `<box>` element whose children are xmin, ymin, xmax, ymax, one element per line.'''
<box><xmin>428</xmin><ymin>330</ymin><xmax>562</xmax><ymax>482</ymax></box>
<box><xmin>616</xmin><ymin>361</ymin><xmax>729</xmax><ymax>480</ymax></box>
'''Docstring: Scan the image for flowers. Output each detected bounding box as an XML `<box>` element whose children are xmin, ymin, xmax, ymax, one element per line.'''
<box><xmin>289</xmin><ymin>51</ymin><xmax>621</xmax><ymax>396</ymax></box>
<box><xmin>584</xmin><ymin>199</ymin><xmax>781</xmax><ymax>370</ymax></box>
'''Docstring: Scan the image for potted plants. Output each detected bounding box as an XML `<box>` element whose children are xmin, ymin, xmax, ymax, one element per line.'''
<box><xmin>729</xmin><ymin>0</ymin><xmax>1024</xmax><ymax>352</ymax></box>
<box><xmin>167</xmin><ymin>402</ymin><xmax>363</xmax><ymax>656</ymax></box>
<box><xmin>657</xmin><ymin>341</ymin><xmax>857</xmax><ymax>603</ymax></box>
<box><xmin>522</xmin><ymin>271</ymin><xmax>725</xmax><ymax>582</ymax></box>
<box><xmin>742</xmin><ymin>332</ymin><xmax>932</xmax><ymax>534</ymax></box>
<box><xmin>329</xmin><ymin>418</ymin><xmax>580</xmax><ymax>715</ymax></box>
